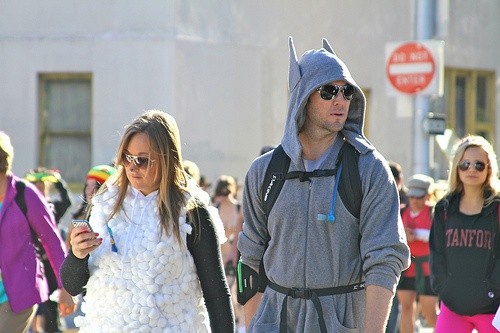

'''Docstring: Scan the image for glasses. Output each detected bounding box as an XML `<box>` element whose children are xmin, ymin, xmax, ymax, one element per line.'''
<box><xmin>316</xmin><ymin>84</ymin><xmax>354</xmax><ymax>100</ymax></box>
<box><xmin>458</xmin><ymin>160</ymin><xmax>487</xmax><ymax>171</ymax></box>
<box><xmin>121</xmin><ymin>151</ymin><xmax>156</xmax><ymax>170</ymax></box>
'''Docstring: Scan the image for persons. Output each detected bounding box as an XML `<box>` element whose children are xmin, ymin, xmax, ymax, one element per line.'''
<box><xmin>23</xmin><ymin>161</ymin><xmax>451</xmax><ymax>333</ymax></box>
<box><xmin>428</xmin><ymin>133</ymin><xmax>500</xmax><ymax>333</ymax></box>
<box><xmin>0</xmin><ymin>132</ymin><xmax>77</xmax><ymax>333</ymax></box>
<box><xmin>58</xmin><ymin>109</ymin><xmax>236</xmax><ymax>333</ymax></box>
<box><xmin>235</xmin><ymin>36</ymin><xmax>411</xmax><ymax>333</ymax></box>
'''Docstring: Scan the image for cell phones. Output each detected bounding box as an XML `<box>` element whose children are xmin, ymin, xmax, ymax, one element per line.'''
<box><xmin>72</xmin><ymin>220</ymin><xmax>98</xmax><ymax>246</ymax></box>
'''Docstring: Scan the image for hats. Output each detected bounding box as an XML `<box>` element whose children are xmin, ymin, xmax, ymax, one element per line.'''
<box><xmin>85</xmin><ymin>164</ymin><xmax>118</xmax><ymax>182</ymax></box>
<box><xmin>405</xmin><ymin>173</ymin><xmax>433</xmax><ymax>196</ymax></box>
